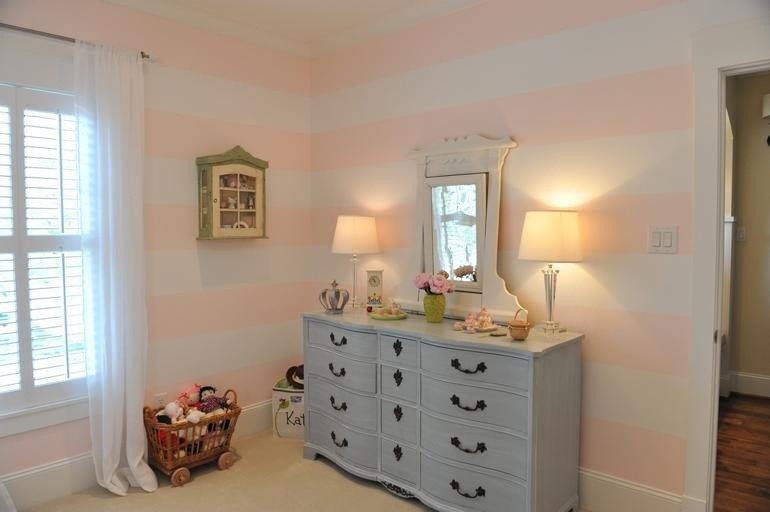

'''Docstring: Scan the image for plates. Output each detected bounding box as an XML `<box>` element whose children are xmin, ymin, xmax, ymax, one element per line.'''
<box><xmin>368</xmin><ymin>311</ymin><xmax>409</xmax><ymax>321</ymax></box>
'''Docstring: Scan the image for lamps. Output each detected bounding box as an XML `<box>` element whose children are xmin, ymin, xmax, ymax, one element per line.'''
<box><xmin>517</xmin><ymin>210</ymin><xmax>583</xmax><ymax>333</ymax></box>
<box><xmin>762</xmin><ymin>94</ymin><xmax>770</xmax><ymax>146</ymax></box>
<box><xmin>331</xmin><ymin>215</ymin><xmax>379</xmax><ymax>308</ymax></box>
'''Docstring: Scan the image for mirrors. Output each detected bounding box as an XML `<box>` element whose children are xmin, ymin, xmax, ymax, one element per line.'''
<box><xmin>423</xmin><ymin>174</ymin><xmax>486</xmax><ymax>293</ymax></box>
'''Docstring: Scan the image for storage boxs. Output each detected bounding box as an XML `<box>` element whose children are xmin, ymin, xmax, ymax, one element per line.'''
<box><xmin>272</xmin><ymin>377</ymin><xmax>304</xmax><ymax>442</ymax></box>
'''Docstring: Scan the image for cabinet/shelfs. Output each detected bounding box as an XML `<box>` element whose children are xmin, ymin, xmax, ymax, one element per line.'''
<box><xmin>301</xmin><ymin>307</ymin><xmax>584</xmax><ymax>512</ymax></box>
<box><xmin>196</xmin><ymin>145</ymin><xmax>269</xmax><ymax>240</ymax></box>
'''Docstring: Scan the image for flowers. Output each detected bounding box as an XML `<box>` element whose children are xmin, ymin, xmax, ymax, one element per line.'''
<box><xmin>454</xmin><ymin>265</ymin><xmax>473</xmax><ymax>279</ymax></box>
<box><xmin>412</xmin><ymin>269</ymin><xmax>456</xmax><ymax>295</ymax></box>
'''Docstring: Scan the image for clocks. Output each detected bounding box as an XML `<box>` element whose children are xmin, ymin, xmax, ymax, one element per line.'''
<box><xmin>368</xmin><ymin>276</ymin><xmax>380</xmax><ymax>288</ymax></box>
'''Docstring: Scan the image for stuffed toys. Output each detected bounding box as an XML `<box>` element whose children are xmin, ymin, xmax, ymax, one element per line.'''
<box><xmin>219</xmin><ymin>175</ymin><xmax>254</xmax><ymax>209</ymax></box>
<box><xmin>155</xmin><ymin>383</ymin><xmax>231</xmax><ymax>458</ymax></box>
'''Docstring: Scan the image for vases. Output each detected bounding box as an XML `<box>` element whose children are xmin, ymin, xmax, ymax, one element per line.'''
<box><xmin>423</xmin><ymin>294</ymin><xmax>446</xmax><ymax>323</ymax></box>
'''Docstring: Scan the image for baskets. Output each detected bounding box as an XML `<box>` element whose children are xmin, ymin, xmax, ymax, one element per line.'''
<box><xmin>143</xmin><ymin>389</ymin><xmax>241</xmax><ymax>486</ymax></box>
